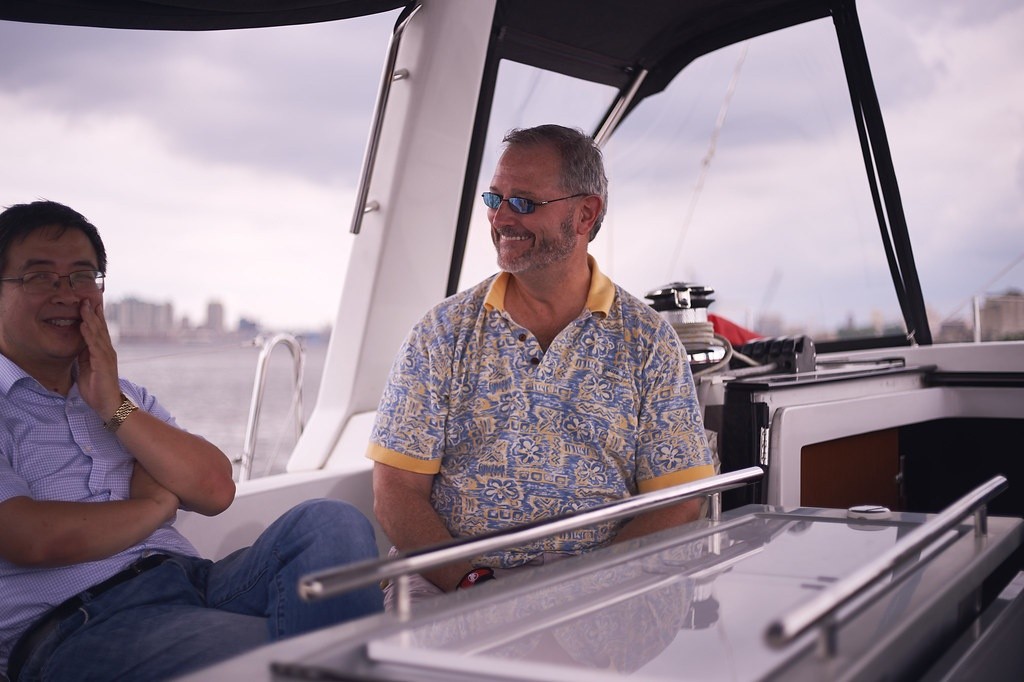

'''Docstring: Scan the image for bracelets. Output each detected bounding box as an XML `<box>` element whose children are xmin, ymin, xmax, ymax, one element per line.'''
<box><xmin>456</xmin><ymin>568</ymin><xmax>494</xmax><ymax>592</ymax></box>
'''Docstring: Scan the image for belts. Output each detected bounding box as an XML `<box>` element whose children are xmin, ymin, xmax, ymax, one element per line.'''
<box><xmin>6</xmin><ymin>553</ymin><xmax>168</xmax><ymax>682</ymax></box>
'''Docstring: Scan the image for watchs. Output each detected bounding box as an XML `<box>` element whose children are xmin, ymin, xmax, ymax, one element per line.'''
<box><xmin>103</xmin><ymin>392</ymin><xmax>139</xmax><ymax>434</ymax></box>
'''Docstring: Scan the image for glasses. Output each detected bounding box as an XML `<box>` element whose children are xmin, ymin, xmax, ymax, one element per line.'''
<box><xmin>481</xmin><ymin>191</ymin><xmax>590</xmax><ymax>214</ymax></box>
<box><xmin>0</xmin><ymin>269</ymin><xmax>106</xmax><ymax>295</ymax></box>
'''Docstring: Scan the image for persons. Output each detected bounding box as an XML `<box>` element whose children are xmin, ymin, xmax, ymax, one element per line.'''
<box><xmin>0</xmin><ymin>201</ymin><xmax>384</xmax><ymax>682</ymax></box>
<box><xmin>405</xmin><ymin>541</ymin><xmax>705</xmax><ymax>673</ymax></box>
<box><xmin>366</xmin><ymin>124</ymin><xmax>716</xmax><ymax>612</ymax></box>
